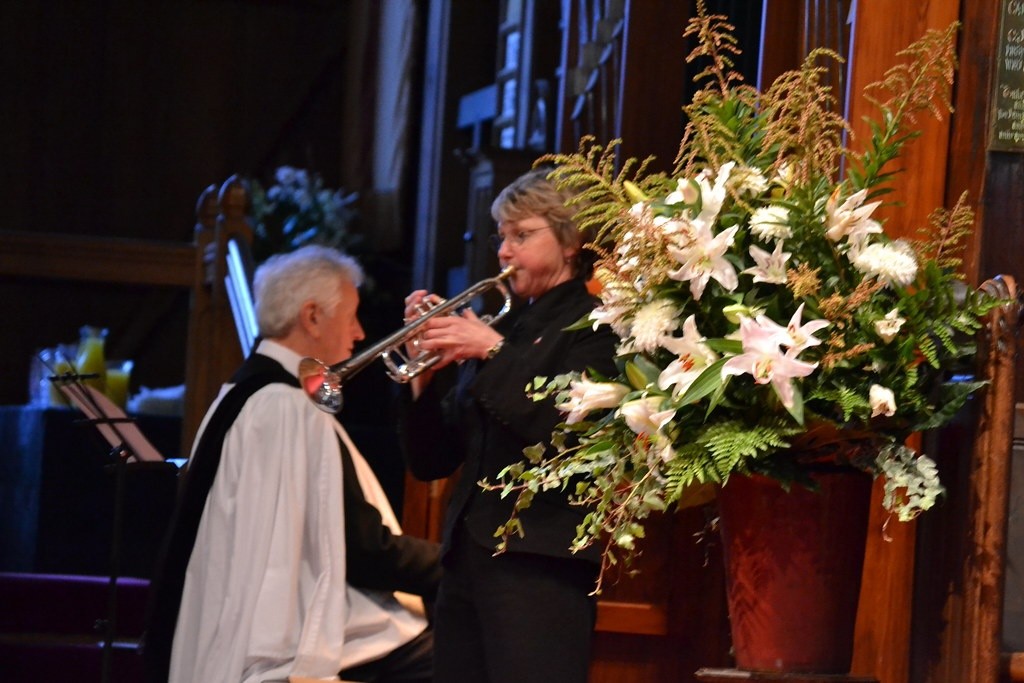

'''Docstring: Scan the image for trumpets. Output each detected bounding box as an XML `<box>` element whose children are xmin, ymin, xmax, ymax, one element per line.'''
<box><xmin>300</xmin><ymin>264</ymin><xmax>520</xmax><ymax>415</ymax></box>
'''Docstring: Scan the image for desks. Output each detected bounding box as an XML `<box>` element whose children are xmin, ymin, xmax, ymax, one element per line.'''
<box><xmin>0</xmin><ymin>406</ymin><xmax>181</xmax><ymax>579</ymax></box>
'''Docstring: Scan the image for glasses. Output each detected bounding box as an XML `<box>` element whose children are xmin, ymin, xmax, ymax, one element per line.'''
<box><xmin>487</xmin><ymin>226</ymin><xmax>553</xmax><ymax>249</ymax></box>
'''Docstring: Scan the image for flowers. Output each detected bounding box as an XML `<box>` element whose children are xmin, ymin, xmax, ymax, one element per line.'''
<box><xmin>478</xmin><ymin>0</ymin><xmax>1023</xmax><ymax>599</ymax></box>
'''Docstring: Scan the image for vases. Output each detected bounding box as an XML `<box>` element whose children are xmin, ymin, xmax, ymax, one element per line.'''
<box><xmin>716</xmin><ymin>463</ymin><xmax>866</xmax><ymax>677</ymax></box>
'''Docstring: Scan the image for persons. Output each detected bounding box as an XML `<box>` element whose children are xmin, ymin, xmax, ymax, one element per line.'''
<box><xmin>399</xmin><ymin>169</ymin><xmax>618</xmax><ymax>683</ymax></box>
<box><xmin>169</xmin><ymin>245</ymin><xmax>439</xmax><ymax>683</ymax></box>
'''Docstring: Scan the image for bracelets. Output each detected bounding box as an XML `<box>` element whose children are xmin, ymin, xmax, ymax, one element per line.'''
<box><xmin>487</xmin><ymin>340</ymin><xmax>502</xmax><ymax>359</ymax></box>
